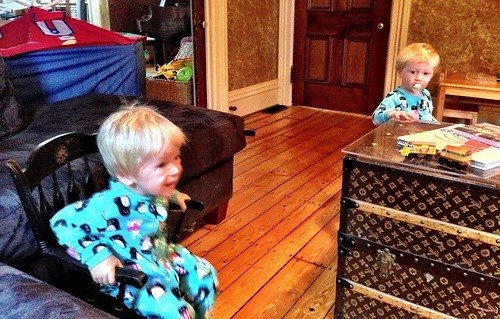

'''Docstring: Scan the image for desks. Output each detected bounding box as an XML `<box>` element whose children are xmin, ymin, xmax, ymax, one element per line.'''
<box><xmin>434</xmin><ymin>72</ymin><xmax>500</xmax><ymax>122</ymax></box>
<box><xmin>334</xmin><ymin>116</ymin><xmax>500</xmax><ymax>319</ymax></box>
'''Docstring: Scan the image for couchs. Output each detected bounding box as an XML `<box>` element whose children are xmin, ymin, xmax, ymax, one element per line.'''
<box><xmin>0</xmin><ymin>56</ymin><xmax>246</xmax><ymax>319</ymax></box>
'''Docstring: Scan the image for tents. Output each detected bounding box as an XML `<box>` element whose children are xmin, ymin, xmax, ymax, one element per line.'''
<box><xmin>0</xmin><ymin>5</ymin><xmax>148</xmax><ymax>114</ymax></box>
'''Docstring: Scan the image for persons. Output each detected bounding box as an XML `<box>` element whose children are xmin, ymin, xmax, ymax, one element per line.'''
<box><xmin>371</xmin><ymin>43</ymin><xmax>440</xmax><ymax>126</ymax></box>
<box><xmin>49</xmin><ymin>101</ymin><xmax>220</xmax><ymax>319</ymax></box>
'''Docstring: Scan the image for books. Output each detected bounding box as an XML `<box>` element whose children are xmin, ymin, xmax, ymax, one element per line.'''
<box><xmin>396</xmin><ymin>121</ymin><xmax>500</xmax><ymax>171</ymax></box>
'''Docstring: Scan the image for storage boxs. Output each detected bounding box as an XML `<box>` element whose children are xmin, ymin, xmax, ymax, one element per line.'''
<box><xmin>146</xmin><ymin>78</ymin><xmax>192</xmax><ymax>105</ymax></box>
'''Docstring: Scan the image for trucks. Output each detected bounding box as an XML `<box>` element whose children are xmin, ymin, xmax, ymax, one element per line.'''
<box><xmin>438</xmin><ymin>145</ymin><xmax>472</xmax><ymax>170</ymax></box>
<box><xmin>400</xmin><ymin>141</ymin><xmax>437</xmax><ymax>161</ymax></box>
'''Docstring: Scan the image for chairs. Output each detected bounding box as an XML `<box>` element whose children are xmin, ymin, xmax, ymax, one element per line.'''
<box><xmin>436</xmin><ymin>73</ymin><xmax>478</xmax><ymax>126</ymax></box>
<box><xmin>5</xmin><ymin>131</ymin><xmax>205</xmax><ymax>319</ymax></box>
